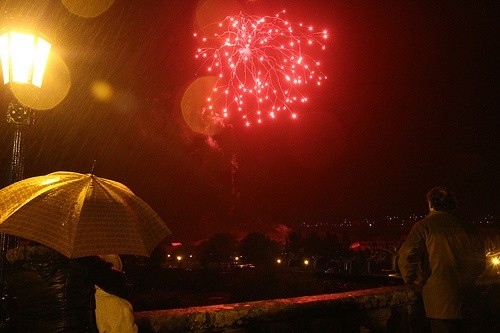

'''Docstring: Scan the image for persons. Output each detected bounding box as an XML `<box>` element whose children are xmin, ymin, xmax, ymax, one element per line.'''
<box><xmin>62</xmin><ymin>253</ymin><xmax>125</xmax><ymax>332</ymax></box>
<box><xmin>398</xmin><ymin>186</ymin><xmax>488</xmax><ymax>332</ymax></box>
<box><xmin>94</xmin><ymin>251</ymin><xmax>140</xmax><ymax>332</ymax></box>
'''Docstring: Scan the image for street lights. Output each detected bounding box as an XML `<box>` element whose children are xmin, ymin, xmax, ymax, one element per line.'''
<box><xmin>3</xmin><ymin>23</ymin><xmax>59</xmax><ymax>272</ymax></box>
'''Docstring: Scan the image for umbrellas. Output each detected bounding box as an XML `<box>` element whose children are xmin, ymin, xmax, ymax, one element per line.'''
<box><xmin>1</xmin><ymin>158</ymin><xmax>172</xmax><ymax>259</ymax></box>
